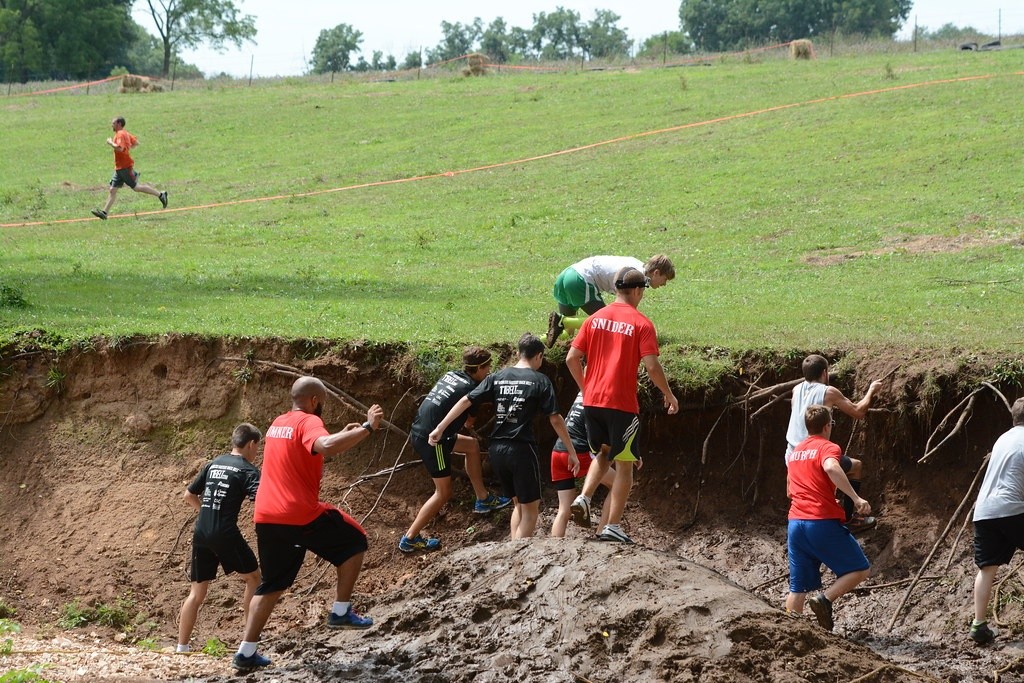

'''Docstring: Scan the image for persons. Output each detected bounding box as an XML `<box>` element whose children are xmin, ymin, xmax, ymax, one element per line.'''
<box><xmin>231</xmin><ymin>376</ymin><xmax>384</xmax><ymax>670</ymax></box>
<box><xmin>966</xmin><ymin>397</ymin><xmax>1024</xmax><ymax>642</ymax></box>
<box><xmin>566</xmin><ymin>267</ymin><xmax>679</xmax><ymax>542</ymax></box>
<box><xmin>177</xmin><ymin>422</ymin><xmax>262</xmax><ymax>653</ymax></box>
<box><xmin>536</xmin><ymin>253</ymin><xmax>675</xmax><ymax>348</ymax></box>
<box><xmin>784</xmin><ymin>355</ymin><xmax>883</xmax><ymax>534</ymax></box>
<box><xmin>399</xmin><ymin>346</ymin><xmax>511</xmax><ymax>552</ymax></box>
<box><xmin>428</xmin><ymin>332</ymin><xmax>580</xmax><ymax>540</ymax></box>
<box><xmin>91</xmin><ymin>116</ymin><xmax>168</xmax><ymax>220</ymax></box>
<box><xmin>551</xmin><ymin>367</ymin><xmax>643</xmax><ymax>537</ymax></box>
<box><xmin>786</xmin><ymin>405</ymin><xmax>871</xmax><ymax>631</ymax></box>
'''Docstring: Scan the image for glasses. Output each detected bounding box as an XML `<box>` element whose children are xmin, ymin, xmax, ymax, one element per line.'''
<box><xmin>828</xmin><ymin>420</ymin><xmax>836</xmax><ymax>426</ymax></box>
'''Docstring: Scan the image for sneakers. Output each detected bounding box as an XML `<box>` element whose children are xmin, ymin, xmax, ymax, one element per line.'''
<box><xmin>971</xmin><ymin>620</ymin><xmax>997</xmax><ymax>642</ymax></box>
<box><xmin>231</xmin><ymin>651</ymin><xmax>274</xmax><ymax>670</ymax></box>
<box><xmin>809</xmin><ymin>593</ymin><xmax>832</xmax><ymax>630</ymax></box>
<box><xmin>545</xmin><ymin>310</ymin><xmax>564</xmax><ymax>348</ymax></box>
<box><xmin>92</xmin><ymin>209</ymin><xmax>107</xmax><ymax>219</ymax></box>
<box><xmin>328</xmin><ymin>606</ymin><xmax>373</xmax><ymax>629</ymax></box>
<box><xmin>600</xmin><ymin>526</ymin><xmax>633</xmax><ymax>542</ymax></box>
<box><xmin>845</xmin><ymin>515</ymin><xmax>877</xmax><ymax>535</ymax></box>
<box><xmin>159</xmin><ymin>190</ymin><xmax>167</xmax><ymax>207</ymax></box>
<box><xmin>571</xmin><ymin>493</ymin><xmax>592</xmax><ymax>528</ymax></box>
<box><xmin>398</xmin><ymin>534</ymin><xmax>440</xmax><ymax>553</ymax></box>
<box><xmin>475</xmin><ymin>491</ymin><xmax>511</xmax><ymax>512</ymax></box>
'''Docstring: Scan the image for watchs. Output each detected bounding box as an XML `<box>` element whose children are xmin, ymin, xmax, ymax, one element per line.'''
<box><xmin>362</xmin><ymin>422</ymin><xmax>373</xmax><ymax>435</ymax></box>
